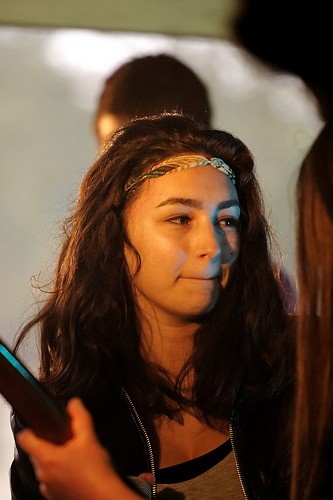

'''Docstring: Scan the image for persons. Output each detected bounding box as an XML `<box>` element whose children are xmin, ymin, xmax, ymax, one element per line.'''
<box><xmin>18</xmin><ymin>121</ymin><xmax>332</xmax><ymax>500</ymax></box>
<box><xmin>95</xmin><ymin>50</ymin><xmax>300</xmax><ymax>318</ymax></box>
<box><xmin>10</xmin><ymin>110</ymin><xmax>332</xmax><ymax>500</ymax></box>
